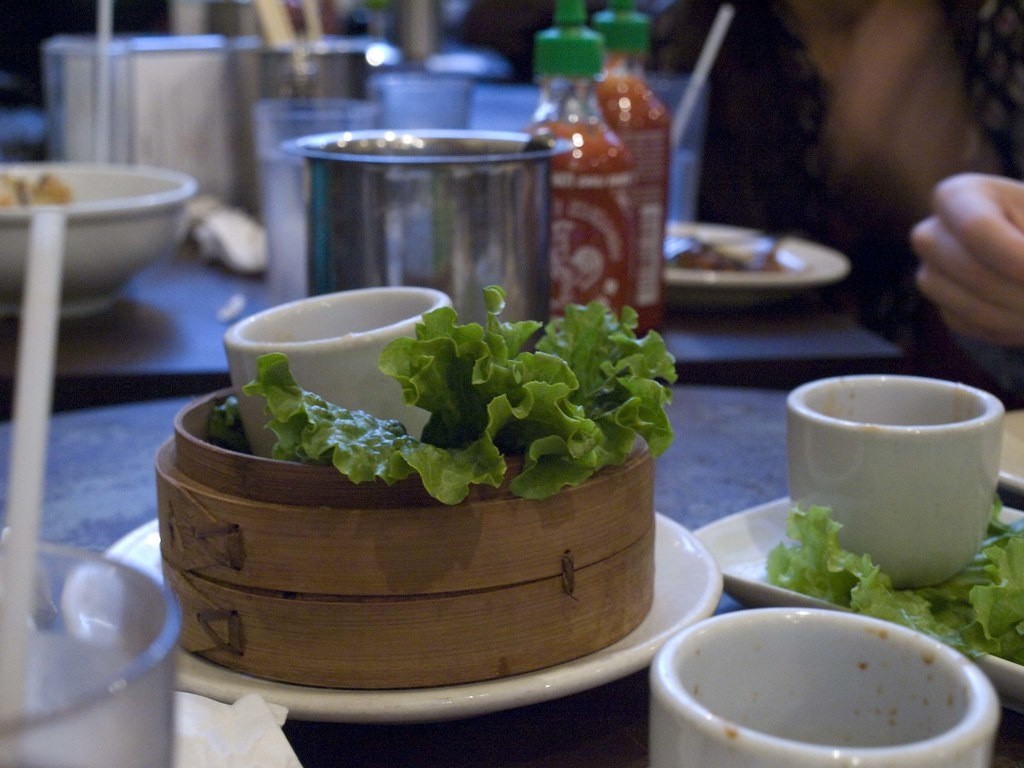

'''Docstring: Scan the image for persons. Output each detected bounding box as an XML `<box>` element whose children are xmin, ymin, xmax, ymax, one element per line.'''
<box><xmin>909</xmin><ymin>172</ymin><xmax>1024</xmax><ymax>352</ymax></box>
<box><xmin>700</xmin><ymin>1</ymin><xmax>1023</xmax><ymax>379</ymax></box>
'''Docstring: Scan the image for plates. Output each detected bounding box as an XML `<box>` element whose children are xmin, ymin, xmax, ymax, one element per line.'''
<box><xmin>999</xmin><ymin>410</ymin><xmax>1024</xmax><ymax>493</ymax></box>
<box><xmin>173</xmin><ymin>692</ymin><xmax>299</xmax><ymax>768</ymax></box>
<box><xmin>662</xmin><ymin>220</ymin><xmax>850</xmax><ymax>308</ymax></box>
<box><xmin>692</xmin><ymin>497</ymin><xmax>1024</xmax><ymax>715</ymax></box>
<box><xmin>103</xmin><ymin>514</ymin><xmax>723</xmax><ymax>719</ymax></box>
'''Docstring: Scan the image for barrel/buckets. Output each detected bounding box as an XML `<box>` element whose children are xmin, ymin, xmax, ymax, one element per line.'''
<box><xmin>276</xmin><ymin>129</ymin><xmax>572</xmax><ymax>326</ymax></box>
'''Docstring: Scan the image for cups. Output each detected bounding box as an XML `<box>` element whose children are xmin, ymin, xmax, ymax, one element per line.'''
<box><xmin>0</xmin><ymin>543</ymin><xmax>183</xmax><ymax>768</ymax></box>
<box><xmin>650</xmin><ymin>76</ymin><xmax>710</xmax><ymax>225</ymax></box>
<box><xmin>368</xmin><ymin>74</ymin><xmax>471</xmax><ymax>137</ymax></box>
<box><xmin>251</xmin><ymin>98</ymin><xmax>379</xmax><ymax>294</ymax></box>
<box><xmin>476</xmin><ymin>84</ymin><xmax>539</xmax><ymax>134</ymax></box>
<box><xmin>223</xmin><ymin>287</ymin><xmax>452</xmax><ymax>456</ymax></box>
<box><xmin>786</xmin><ymin>373</ymin><xmax>1006</xmax><ymax>586</ymax></box>
<box><xmin>649</xmin><ymin>608</ymin><xmax>1000</xmax><ymax>768</ymax></box>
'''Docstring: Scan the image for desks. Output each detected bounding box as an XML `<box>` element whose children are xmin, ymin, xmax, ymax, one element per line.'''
<box><xmin>0</xmin><ymin>249</ymin><xmax>1024</xmax><ymax>768</ymax></box>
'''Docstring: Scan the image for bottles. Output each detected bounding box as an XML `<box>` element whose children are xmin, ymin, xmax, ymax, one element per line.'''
<box><xmin>525</xmin><ymin>0</ymin><xmax>635</xmax><ymax>322</ymax></box>
<box><xmin>593</xmin><ymin>0</ymin><xmax>671</xmax><ymax>335</ymax></box>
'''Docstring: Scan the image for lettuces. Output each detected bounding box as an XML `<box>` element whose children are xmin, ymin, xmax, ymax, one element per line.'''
<box><xmin>206</xmin><ymin>285</ymin><xmax>677</xmax><ymax>506</ymax></box>
<box><xmin>762</xmin><ymin>490</ymin><xmax>1024</xmax><ymax>668</ymax></box>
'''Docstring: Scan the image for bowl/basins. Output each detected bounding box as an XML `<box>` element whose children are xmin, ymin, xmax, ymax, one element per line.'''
<box><xmin>0</xmin><ymin>164</ymin><xmax>198</xmax><ymax>317</ymax></box>
